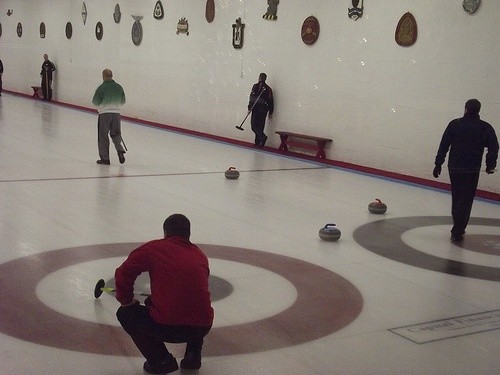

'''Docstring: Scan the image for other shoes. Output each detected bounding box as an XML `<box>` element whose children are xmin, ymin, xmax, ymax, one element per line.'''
<box><xmin>119</xmin><ymin>151</ymin><xmax>125</xmax><ymax>164</ymax></box>
<box><xmin>451</xmin><ymin>235</ymin><xmax>464</xmax><ymax>241</ymax></box>
<box><xmin>97</xmin><ymin>160</ymin><xmax>110</xmax><ymax>165</ymax></box>
<box><xmin>143</xmin><ymin>353</ymin><xmax>179</xmax><ymax>374</ymax></box>
<box><xmin>180</xmin><ymin>354</ymin><xmax>201</xmax><ymax>369</ymax></box>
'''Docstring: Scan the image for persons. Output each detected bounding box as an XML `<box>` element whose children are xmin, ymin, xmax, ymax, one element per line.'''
<box><xmin>92</xmin><ymin>68</ymin><xmax>128</xmax><ymax>165</ymax></box>
<box><xmin>40</xmin><ymin>53</ymin><xmax>55</xmax><ymax>102</ymax></box>
<box><xmin>432</xmin><ymin>99</ymin><xmax>499</xmax><ymax>241</ymax></box>
<box><xmin>248</xmin><ymin>73</ymin><xmax>274</xmax><ymax>148</ymax></box>
<box><xmin>0</xmin><ymin>60</ymin><xmax>4</xmax><ymax>97</ymax></box>
<box><xmin>115</xmin><ymin>212</ymin><xmax>214</xmax><ymax>375</ymax></box>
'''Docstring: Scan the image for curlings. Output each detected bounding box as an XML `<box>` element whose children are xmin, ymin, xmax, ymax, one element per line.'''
<box><xmin>368</xmin><ymin>196</ymin><xmax>387</xmax><ymax>215</ymax></box>
<box><xmin>224</xmin><ymin>166</ymin><xmax>241</xmax><ymax>181</ymax></box>
<box><xmin>318</xmin><ymin>221</ymin><xmax>342</xmax><ymax>242</ymax></box>
